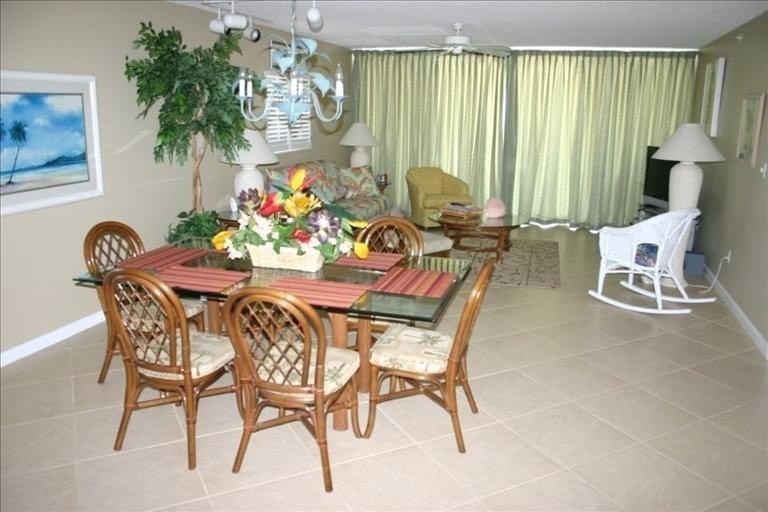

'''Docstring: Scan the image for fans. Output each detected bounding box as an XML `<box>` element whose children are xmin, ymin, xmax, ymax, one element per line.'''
<box><xmin>416</xmin><ymin>23</ymin><xmax>512</xmax><ymax>58</ymax></box>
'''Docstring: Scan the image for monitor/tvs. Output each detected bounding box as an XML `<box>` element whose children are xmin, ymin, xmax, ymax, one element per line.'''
<box><xmin>642</xmin><ymin>146</ymin><xmax>680</xmax><ymax>210</ymax></box>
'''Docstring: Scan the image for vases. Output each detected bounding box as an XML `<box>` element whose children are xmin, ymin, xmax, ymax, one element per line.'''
<box><xmin>247</xmin><ymin>240</ymin><xmax>324</xmax><ymax>272</ymax></box>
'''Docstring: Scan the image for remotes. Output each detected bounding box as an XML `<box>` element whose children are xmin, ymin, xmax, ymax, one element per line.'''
<box><xmin>450</xmin><ymin>201</ymin><xmax>466</xmax><ymax>206</ymax></box>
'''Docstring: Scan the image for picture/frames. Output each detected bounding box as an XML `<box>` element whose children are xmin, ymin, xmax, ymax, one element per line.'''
<box><xmin>0</xmin><ymin>69</ymin><xmax>104</xmax><ymax>218</ymax></box>
<box><xmin>699</xmin><ymin>57</ymin><xmax>726</xmax><ymax>137</ymax></box>
<box><xmin>735</xmin><ymin>92</ymin><xmax>766</xmax><ymax>168</ymax></box>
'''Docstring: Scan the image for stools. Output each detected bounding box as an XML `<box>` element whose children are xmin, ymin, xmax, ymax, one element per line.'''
<box><xmin>419</xmin><ymin>230</ymin><xmax>454</xmax><ymax>258</ymax></box>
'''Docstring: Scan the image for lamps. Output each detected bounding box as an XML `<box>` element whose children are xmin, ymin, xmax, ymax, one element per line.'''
<box><xmin>208</xmin><ymin>0</ymin><xmax>350</xmax><ymax>128</ymax></box>
<box><xmin>650</xmin><ymin>124</ymin><xmax>726</xmax><ymax>251</ymax></box>
<box><xmin>217</xmin><ymin>128</ymin><xmax>279</xmax><ymax>199</ymax></box>
<box><xmin>338</xmin><ymin>124</ymin><xmax>378</xmax><ymax>170</ymax></box>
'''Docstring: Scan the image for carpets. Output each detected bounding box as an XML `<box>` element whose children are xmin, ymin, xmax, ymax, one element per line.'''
<box><xmin>446</xmin><ymin>237</ymin><xmax>562</xmax><ymax>290</ymax></box>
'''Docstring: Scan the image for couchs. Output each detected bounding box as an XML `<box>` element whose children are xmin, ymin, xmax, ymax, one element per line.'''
<box><xmin>266</xmin><ymin>160</ymin><xmax>394</xmax><ymax>221</ymax></box>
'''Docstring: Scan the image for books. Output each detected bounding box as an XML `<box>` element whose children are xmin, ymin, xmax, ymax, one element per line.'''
<box><xmin>439</xmin><ymin>204</ymin><xmax>480</xmax><ymax>219</ymax></box>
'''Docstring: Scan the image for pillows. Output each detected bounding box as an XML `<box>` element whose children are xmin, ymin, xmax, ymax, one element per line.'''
<box><xmin>339</xmin><ymin>166</ymin><xmax>382</xmax><ymax>196</ymax></box>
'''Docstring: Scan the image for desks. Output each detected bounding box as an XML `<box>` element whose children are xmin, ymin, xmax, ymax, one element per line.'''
<box><xmin>637</xmin><ymin>205</ymin><xmax>664</xmax><ymax>225</ymax></box>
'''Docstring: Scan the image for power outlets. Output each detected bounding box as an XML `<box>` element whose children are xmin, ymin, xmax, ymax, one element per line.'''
<box><xmin>726</xmin><ymin>249</ymin><xmax>732</xmax><ymax>264</ymax></box>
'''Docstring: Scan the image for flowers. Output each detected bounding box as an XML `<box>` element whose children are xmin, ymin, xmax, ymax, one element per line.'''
<box><xmin>211</xmin><ymin>164</ymin><xmax>368</xmax><ymax>263</ymax></box>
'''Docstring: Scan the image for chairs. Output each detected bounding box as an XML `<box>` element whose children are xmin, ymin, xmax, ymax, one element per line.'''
<box><xmin>405</xmin><ymin>166</ymin><xmax>472</xmax><ymax>232</ymax></box>
<box><xmin>588</xmin><ymin>208</ymin><xmax>715</xmax><ymax>315</ymax></box>
